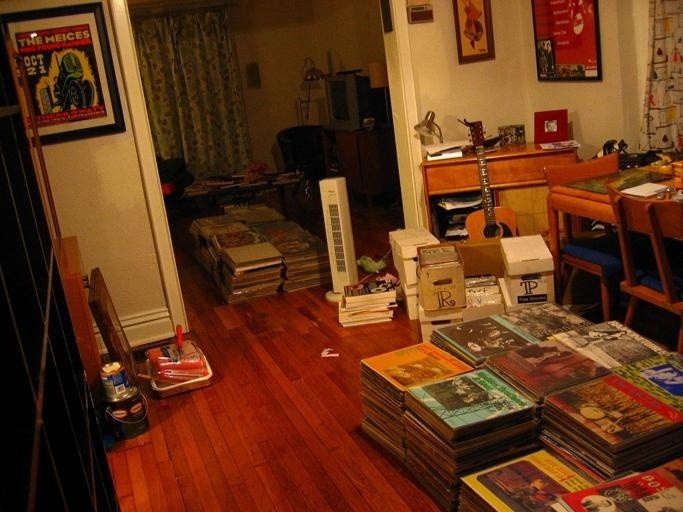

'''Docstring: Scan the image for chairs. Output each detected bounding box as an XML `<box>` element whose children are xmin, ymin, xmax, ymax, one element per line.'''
<box><xmin>158</xmin><ymin>157</ymin><xmax>196</xmax><ymax>194</ymax></box>
<box><xmin>544</xmin><ymin>151</ymin><xmax>625</xmax><ymax>322</ymax></box>
<box><xmin>276</xmin><ymin>125</ymin><xmax>325</xmax><ymax>214</ymax></box>
<box><xmin>606</xmin><ymin>183</ymin><xmax>683</xmax><ymax>357</ymax></box>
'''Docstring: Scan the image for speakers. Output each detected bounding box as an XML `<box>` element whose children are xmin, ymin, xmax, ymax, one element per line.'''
<box><xmin>367</xmin><ymin>86</ymin><xmax>390</xmax><ymax>129</ymax></box>
<box><xmin>318</xmin><ymin>175</ymin><xmax>358</xmax><ymax>302</ymax></box>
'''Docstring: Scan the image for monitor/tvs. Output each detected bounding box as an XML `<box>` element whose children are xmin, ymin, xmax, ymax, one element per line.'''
<box><xmin>325</xmin><ymin>74</ymin><xmax>374</xmax><ymax>130</ymax></box>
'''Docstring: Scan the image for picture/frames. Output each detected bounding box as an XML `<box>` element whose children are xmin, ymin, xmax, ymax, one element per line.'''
<box><xmin>0</xmin><ymin>2</ymin><xmax>126</xmax><ymax>148</ymax></box>
<box><xmin>531</xmin><ymin>0</ymin><xmax>602</xmax><ymax>81</ymax></box>
<box><xmin>534</xmin><ymin>108</ymin><xmax>568</xmax><ymax>144</ymax></box>
<box><xmin>452</xmin><ymin>0</ymin><xmax>496</xmax><ymax>65</ymax></box>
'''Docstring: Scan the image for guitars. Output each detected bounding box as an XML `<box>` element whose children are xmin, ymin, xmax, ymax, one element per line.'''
<box><xmin>459</xmin><ymin>120</ymin><xmax>517</xmax><ymax>239</ymax></box>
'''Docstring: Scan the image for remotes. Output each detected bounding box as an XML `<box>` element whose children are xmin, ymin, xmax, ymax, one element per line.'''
<box><xmin>336</xmin><ymin>69</ymin><xmax>362</xmax><ymax>74</ymax></box>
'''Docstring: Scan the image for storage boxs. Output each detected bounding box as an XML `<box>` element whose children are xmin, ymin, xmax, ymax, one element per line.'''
<box><xmin>389</xmin><ymin>228</ymin><xmax>554</xmax><ymax>343</ymax></box>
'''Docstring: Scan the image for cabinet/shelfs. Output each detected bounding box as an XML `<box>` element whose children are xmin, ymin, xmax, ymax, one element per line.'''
<box><xmin>328</xmin><ymin>125</ymin><xmax>383</xmax><ymax>207</ymax></box>
<box><xmin>0</xmin><ymin>33</ymin><xmax>118</xmax><ymax>512</ymax></box>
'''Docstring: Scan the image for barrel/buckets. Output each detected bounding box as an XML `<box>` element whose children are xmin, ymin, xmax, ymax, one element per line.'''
<box><xmin>99</xmin><ymin>387</ymin><xmax>151</xmax><ymax>441</ymax></box>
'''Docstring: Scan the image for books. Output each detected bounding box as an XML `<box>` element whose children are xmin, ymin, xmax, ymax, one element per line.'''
<box><xmin>620</xmin><ymin>182</ymin><xmax>668</xmax><ymax>198</ymax></box>
<box><xmin>434</xmin><ymin>194</ymin><xmax>483</xmax><ymax>240</ymax></box>
<box><xmin>184</xmin><ymin>162</ymin><xmax>330</xmax><ymax>305</ymax></box>
<box><xmin>538</xmin><ymin>140</ymin><xmax>580</xmax><ymax>150</ymax></box>
<box><xmin>422</xmin><ymin>140</ymin><xmax>463</xmax><ymax>162</ymax></box>
<box><xmin>338</xmin><ymin>278</ymin><xmax>683</xmax><ymax>512</ymax></box>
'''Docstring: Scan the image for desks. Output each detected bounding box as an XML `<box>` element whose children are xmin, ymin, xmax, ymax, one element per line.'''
<box><xmin>181</xmin><ymin>173</ymin><xmax>300</xmax><ymax>216</ymax></box>
<box><xmin>420</xmin><ymin>142</ymin><xmax>581</xmax><ymax>236</ymax></box>
<box><xmin>546</xmin><ymin>161</ymin><xmax>683</xmax><ymax>300</ymax></box>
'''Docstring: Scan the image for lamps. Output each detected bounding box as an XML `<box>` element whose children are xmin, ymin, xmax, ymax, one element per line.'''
<box><xmin>414</xmin><ymin>111</ymin><xmax>443</xmax><ymax>144</ymax></box>
<box><xmin>296</xmin><ymin>57</ymin><xmax>326</xmax><ymax>126</ymax></box>
